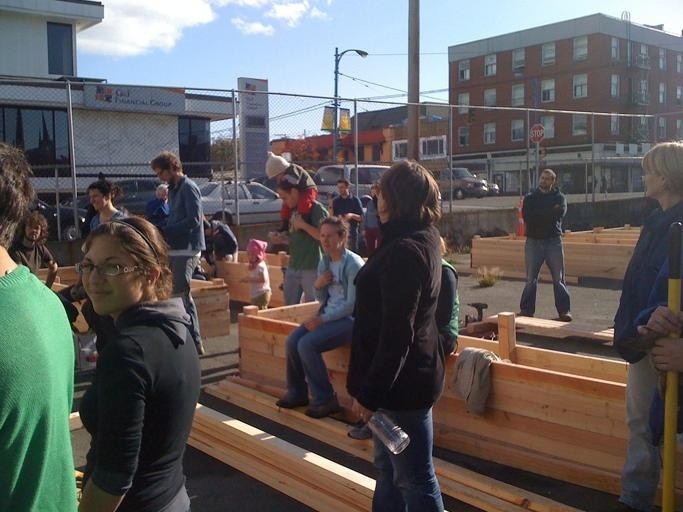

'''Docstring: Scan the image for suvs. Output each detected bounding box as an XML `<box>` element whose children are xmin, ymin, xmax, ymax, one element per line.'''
<box><xmin>428</xmin><ymin>167</ymin><xmax>489</xmax><ymax>203</ymax></box>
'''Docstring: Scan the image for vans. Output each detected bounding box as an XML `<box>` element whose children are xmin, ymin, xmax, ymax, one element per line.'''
<box><xmin>312</xmin><ymin>165</ymin><xmax>393</xmax><ymax>203</ymax></box>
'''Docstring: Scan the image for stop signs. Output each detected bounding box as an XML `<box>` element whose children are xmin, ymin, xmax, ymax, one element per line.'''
<box><xmin>529</xmin><ymin>124</ymin><xmax>546</xmax><ymax>142</ymax></box>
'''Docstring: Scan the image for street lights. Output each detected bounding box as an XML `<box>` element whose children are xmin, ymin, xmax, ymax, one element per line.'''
<box><xmin>332</xmin><ymin>45</ymin><xmax>368</xmax><ymax>164</ymax></box>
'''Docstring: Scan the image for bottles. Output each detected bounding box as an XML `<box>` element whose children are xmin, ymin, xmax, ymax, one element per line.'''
<box><xmin>360</xmin><ymin>406</ymin><xmax>411</xmax><ymax>455</ymax></box>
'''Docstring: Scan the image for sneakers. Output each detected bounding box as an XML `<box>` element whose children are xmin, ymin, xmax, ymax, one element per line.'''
<box><xmin>348</xmin><ymin>419</ymin><xmax>373</xmax><ymax>440</ymax></box>
<box><xmin>276</xmin><ymin>396</ymin><xmax>308</xmax><ymax>409</ymax></box>
<box><xmin>306</xmin><ymin>398</ymin><xmax>341</xmax><ymax>418</ymax></box>
<box><xmin>560</xmin><ymin>312</ymin><xmax>572</xmax><ymax>321</ymax></box>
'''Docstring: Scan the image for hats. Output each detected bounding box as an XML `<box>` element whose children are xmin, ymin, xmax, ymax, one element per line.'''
<box><xmin>247</xmin><ymin>238</ymin><xmax>267</xmax><ymax>260</ymax></box>
<box><xmin>265</xmin><ymin>151</ymin><xmax>291</xmax><ymax>179</ymax></box>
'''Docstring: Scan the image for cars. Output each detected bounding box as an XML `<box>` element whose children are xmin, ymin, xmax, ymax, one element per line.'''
<box><xmin>192</xmin><ymin>181</ymin><xmax>285</xmax><ymax>225</ymax></box>
<box><xmin>58</xmin><ymin>179</ymin><xmax>165</xmax><ymax>216</ymax></box>
<box><xmin>245</xmin><ymin>169</ymin><xmax>317</xmax><ymax>198</ymax></box>
<box><xmin>472</xmin><ymin>173</ymin><xmax>499</xmax><ymax>197</ymax></box>
<box><xmin>32</xmin><ymin>197</ymin><xmax>89</xmax><ymax>242</ymax></box>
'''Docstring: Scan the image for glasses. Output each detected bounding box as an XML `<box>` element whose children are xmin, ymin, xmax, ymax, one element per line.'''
<box><xmin>75</xmin><ymin>262</ymin><xmax>138</xmax><ymax>276</ymax></box>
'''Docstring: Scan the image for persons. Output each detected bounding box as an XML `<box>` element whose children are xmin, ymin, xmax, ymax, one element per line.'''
<box><xmin>330</xmin><ymin>177</ymin><xmax>380</xmax><ymax>262</ymax></box>
<box><xmin>6</xmin><ymin>210</ymin><xmax>79</xmax><ymax>326</ymax></box>
<box><xmin>192</xmin><ymin>216</ymin><xmax>238</xmax><ymax>282</ymax></box>
<box><xmin>57</xmin><ymin>180</ymin><xmax>130</xmax><ymax>358</ymax></box>
<box><xmin>514</xmin><ymin>168</ymin><xmax>574</xmax><ymax>322</ymax></box>
<box><xmin>268</xmin><ymin>180</ymin><xmax>330</xmax><ymax>306</ymax></box>
<box><xmin>143</xmin><ymin>183</ymin><xmax>170</xmax><ymax>241</ymax></box>
<box><xmin>347</xmin><ymin>233</ymin><xmax>462</xmax><ymax>441</ymax></box>
<box><xmin>347</xmin><ymin>159</ymin><xmax>449</xmax><ymax>511</ymax></box>
<box><xmin>273</xmin><ymin>213</ymin><xmax>367</xmax><ymax>420</ymax></box>
<box><xmin>0</xmin><ymin>143</ymin><xmax>84</xmax><ymax>510</ymax></box>
<box><xmin>239</xmin><ymin>238</ymin><xmax>272</xmax><ymax>310</ymax></box>
<box><xmin>266</xmin><ymin>152</ymin><xmax>319</xmax><ymax>233</ymax></box>
<box><xmin>72</xmin><ymin>212</ymin><xmax>204</xmax><ymax>512</ymax></box>
<box><xmin>636</xmin><ymin>305</ymin><xmax>682</xmax><ymax>446</ymax></box>
<box><xmin>151</xmin><ymin>152</ymin><xmax>207</xmax><ymax>358</ymax></box>
<box><xmin>610</xmin><ymin>141</ymin><xmax>682</xmax><ymax>510</ymax></box>
